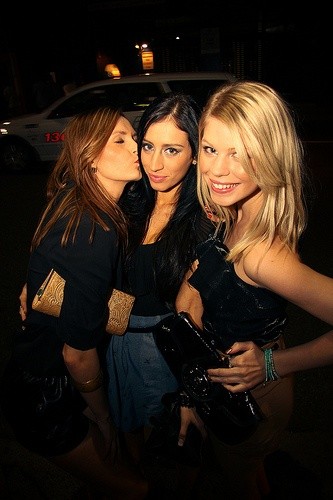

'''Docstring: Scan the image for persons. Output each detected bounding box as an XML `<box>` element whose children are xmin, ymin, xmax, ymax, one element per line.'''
<box><xmin>19</xmin><ymin>92</ymin><xmax>226</xmax><ymax>500</ymax></box>
<box><xmin>173</xmin><ymin>81</ymin><xmax>333</xmax><ymax>500</ymax></box>
<box><xmin>8</xmin><ymin>107</ymin><xmax>143</xmax><ymax>500</ymax></box>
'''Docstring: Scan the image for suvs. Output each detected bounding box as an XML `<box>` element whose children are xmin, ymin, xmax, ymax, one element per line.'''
<box><xmin>0</xmin><ymin>71</ymin><xmax>237</xmax><ymax>176</ymax></box>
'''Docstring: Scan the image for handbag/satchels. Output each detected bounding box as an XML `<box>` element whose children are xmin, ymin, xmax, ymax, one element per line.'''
<box><xmin>30</xmin><ymin>267</ymin><xmax>137</xmax><ymax>336</ymax></box>
<box><xmin>152</xmin><ymin>311</ymin><xmax>262</xmax><ymax>447</ymax></box>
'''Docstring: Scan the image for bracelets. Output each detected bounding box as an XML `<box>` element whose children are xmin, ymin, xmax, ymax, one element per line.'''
<box><xmin>264</xmin><ymin>348</ymin><xmax>280</xmax><ymax>382</ymax></box>
<box><xmin>72</xmin><ymin>372</ymin><xmax>104</xmax><ymax>393</ymax></box>
